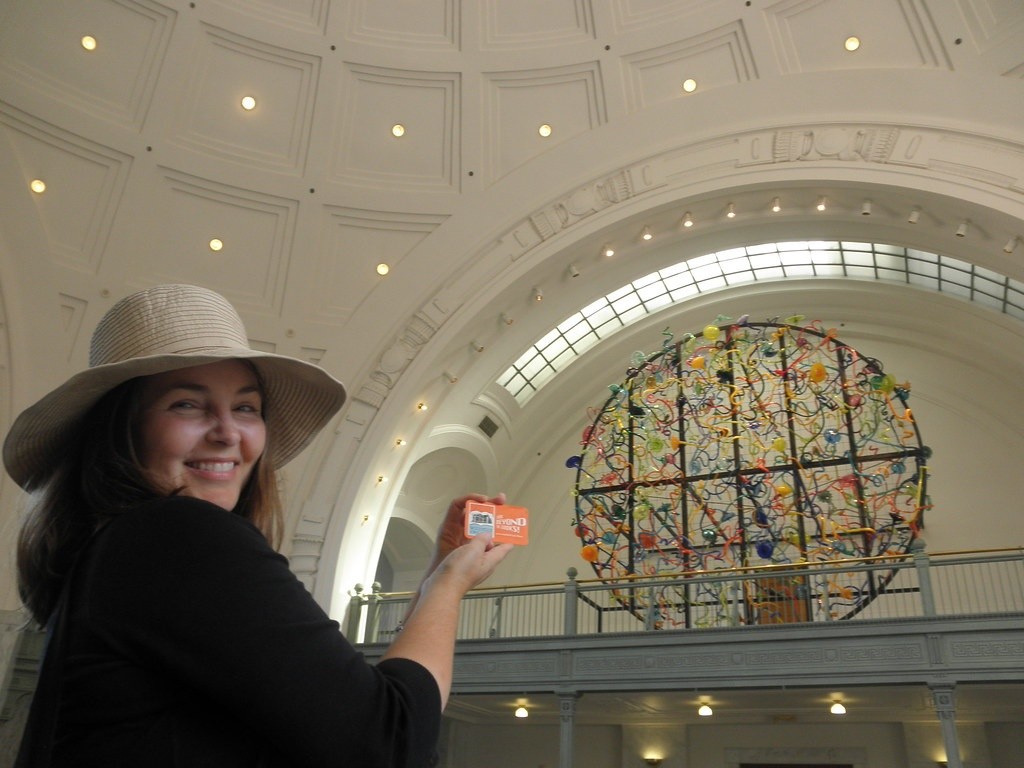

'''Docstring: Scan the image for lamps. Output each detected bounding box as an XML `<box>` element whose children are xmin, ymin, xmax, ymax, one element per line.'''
<box><xmin>644</xmin><ymin>226</ymin><xmax>651</xmax><ymax>239</ymax></box>
<box><xmin>698</xmin><ymin>701</ymin><xmax>713</xmax><ymax>716</ymax></box>
<box><xmin>817</xmin><ymin>196</ymin><xmax>826</xmax><ymax>210</ymax></box>
<box><xmin>772</xmin><ymin>199</ymin><xmax>781</xmax><ymax>211</ymax></box>
<box><xmin>605</xmin><ymin>245</ymin><xmax>613</xmax><ymax>256</ymax></box>
<box><xmin>937</xmin><ymin>761</ymin><xmax>948</xmax><ymax>768</ymax></box>
<box><xmin>830</xmin><ymin>700</ymin><xmax>846</xmax><ymax>714</ymax></box>
<box><xmin>534</xmin><ymin>288</ymin><xmax>543</xmax><ymax>300</ymax></box>
<box><xmin>569</xmin><ymin>263</ymin><xmax>579</xmax><ymax>277</ymax></box>
<box><xmin>955</xmin><ymin>221</ymin><xmax>967</xmax><ymax>237</ymax></box>
<box><xmin>684</xmin><ymin>213</ymin><xmax>693</xmax><ymax>227</ymax></box>
<box><xmin>908</xmin><ymin>210</ymin><xmax>920</xmax><ymax>224</ymax></box>
<box><xmin>861</xmin><ymin>200</ymin><xmax>871</xmax><ymax>215</ymax></box>
<box><xmin>642</xmin><ymin>759</ymin><xmax>662</xmax><ymax>766</ymax></box>
<box><xmin>726</xmin><ymin>204</ymin><xmax>735</xmax><ymax>217</ymax></box>
<box><xmin>514</xmin><ymin>706</ymin><xmax>528</xmax><ymax>717</ymax></box>
<box><xmin>1003</xmin><ymin>237</ymin><xmax>1018</xmax><ymax>253</ymax></box>
<box><xmin>443</xmin><ymin>370</ymin><xmax>457</xmax><ymax>384</ymax></box>
<box><xmin>500</xmin><ymin>313</ymin><xmax>513</xmax><ymax>325</ymax></box>
<box><xmin>471</xmin><ymin>340</ymin><xmax>484</xmax><ymax>352</ymax></box>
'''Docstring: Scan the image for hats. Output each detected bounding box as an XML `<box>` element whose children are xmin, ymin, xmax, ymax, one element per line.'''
<box><xmin>2</xmin><ymin>284</ymin><xmax>348</xmax><ymax>496</ymax></box>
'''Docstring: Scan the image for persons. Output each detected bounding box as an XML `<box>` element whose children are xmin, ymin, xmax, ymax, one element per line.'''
<box><xmin>2</xmin><ymin>284</ymin><xmax>515</xmax><ymax>768</ymax></box>
